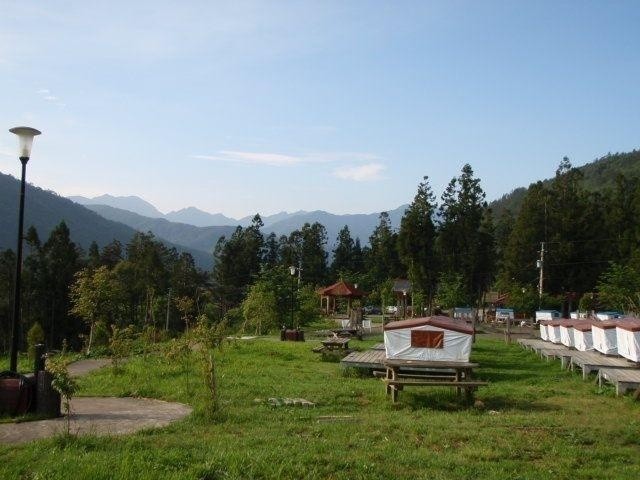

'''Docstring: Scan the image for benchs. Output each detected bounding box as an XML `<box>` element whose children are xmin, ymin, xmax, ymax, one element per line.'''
<box><xmin>373</xmin><ymin>360</ymin><xmax>488</xmax><ymax>403</ymax></box>
<box><xmin>312</xmin><ymin>337</ymin><xmax>355</xmax><ymax>361</ymax></box>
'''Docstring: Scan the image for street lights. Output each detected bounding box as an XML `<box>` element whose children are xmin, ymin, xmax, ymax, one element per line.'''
<box><xmin>4</xmin><ymin>124</ymin><xmax>44</xmax><ymax>375</ymax></box>
<box><xmin>288</xmin><ymin>264</ymin><xmax>298</xmax><ymax>328</ymax></box>
